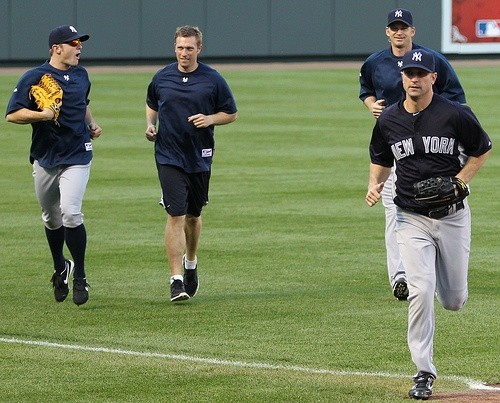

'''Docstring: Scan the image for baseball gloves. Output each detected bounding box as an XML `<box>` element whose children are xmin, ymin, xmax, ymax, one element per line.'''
<box><xmin>30</xmin><ymin>72</ymin><xmax>64</xmax><ymax>122</ymax></box>
<box><xmin>411</xmin><ymin>177</ymin><xmax>470</xmax><ymax>206</ymax></box>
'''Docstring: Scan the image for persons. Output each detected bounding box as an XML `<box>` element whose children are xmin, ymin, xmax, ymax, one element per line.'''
<box><xmin>5</xmin><ymin>25</ymin><xmax>102</xmax><ymax>305</ymax></box>
<box><xmin>144</xmin><ymin>24</ymin><xmax>238</xmax><ymax>301</ymax></box>
<box><xmin>358</xmin><ymin>7</ymin><xmax>466</xmax><ymax>302</ymax></box>
<box><xmin>365</xmin><ymin>49</ymin><xmax>492</xmax><ymax>399</ymax></box>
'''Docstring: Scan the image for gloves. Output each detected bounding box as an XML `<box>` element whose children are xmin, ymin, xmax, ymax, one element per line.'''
<box><xmin>436</xmin><ymin>175</ymin><xmax>469</xmax><ymax>205</ymax></box>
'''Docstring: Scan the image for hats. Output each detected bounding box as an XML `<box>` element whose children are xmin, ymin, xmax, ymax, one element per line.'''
<box><xmin>400</xmin><ymin>49</ymin><xmax>436</xmax><ymax>72</ymax></box>
<box><xmin>386</xmin><ymin>8</ymin><xmax>413</xmax><ymax>28</ymax></box>
<box><xmin>49</xmin><ymin>25</ymin><xmax>90</xmax><ymax>49</ymax></box>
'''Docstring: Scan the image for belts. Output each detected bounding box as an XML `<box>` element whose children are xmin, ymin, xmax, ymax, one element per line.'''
<box><xmin>407</xmin><ymin>202</ymin><xmax>466</xmax><ymax>218</ymax></box>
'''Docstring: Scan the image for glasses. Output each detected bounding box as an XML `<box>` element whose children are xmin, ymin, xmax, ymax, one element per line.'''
<box><xmin>56</xmin><ymin>39</ymin><xmax>82</xmax><ymax>47</ymax></box>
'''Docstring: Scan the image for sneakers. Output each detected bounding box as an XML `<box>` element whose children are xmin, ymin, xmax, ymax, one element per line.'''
<box><xmin>409</xmin><ymin>371</ymin><xmax>435</xmax><ymax>399</ymax></box>
<box><xmin>71</xmin><ymin>273</ymin><xmax>92</xmax><ymax>306</ymax></box>
<box><xmin>181</xmin><ymin>254</ymin><xmax>199</xmax><ymax>297</ymax></box>
<box><xmin>169</xmin><ymin>277</ymin><xmax>190</xmax><ymax>302</ymax></box>
<box><xmin>51</xmin><ymin>259</ymin><xmax>74</xmax><ymax>302</ymax></box>
<box><xmin>392</xmin><ymin>278</ymin><xmax>409</xmax><ymax>300</ymax></box>
